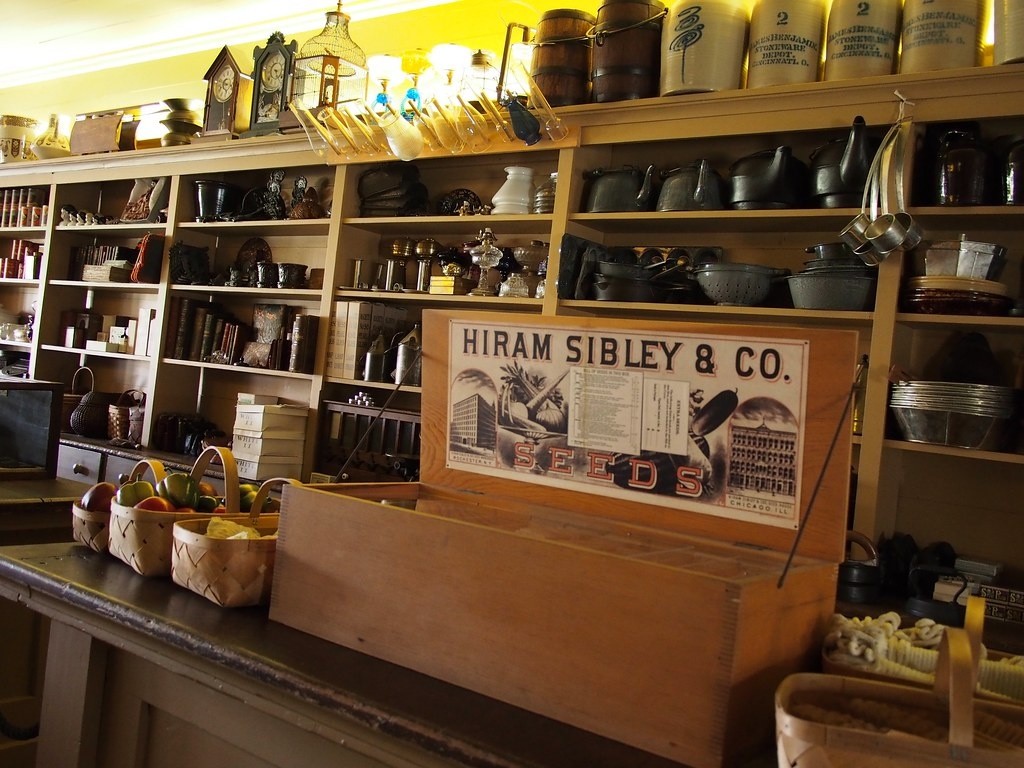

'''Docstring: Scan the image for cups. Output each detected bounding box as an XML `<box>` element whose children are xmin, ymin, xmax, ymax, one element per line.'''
<box><xmin>3</xmin><ymin>323</ymin><xmax>28</xmax><ymax>342</ymax></box>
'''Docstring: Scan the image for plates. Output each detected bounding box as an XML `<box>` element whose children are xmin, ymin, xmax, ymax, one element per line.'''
<box><xmin>906</xmin><ymin>276</ymin><xmax>1010</xmax><ymax>316</ymax></box>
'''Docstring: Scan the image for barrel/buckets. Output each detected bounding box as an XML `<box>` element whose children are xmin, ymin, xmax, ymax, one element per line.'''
<box><xmin>660</xmin><ymin>0</ymin><xmax>754</xmax><ymax>97</ymax></box>
<box><xmin>0</xmin><ymin>114</ymin><xmax>37</xmax><ymax>163</ymax></box>
<box><xmin>994</xmin><ymin>0</ymin><xmax>1024</xmax><ymax>65</ymax></box>
<box><xmin>824</xmin><ymin>0</ymin><xmax>906</xmax><ymax>81</ymax></box>
<box><xmin>586</xmin><ymin>0</ymin><xmax>666</xmax><ymax>103</ymax></box>
<box><xmin>527</xmin><ymin>9</ymin><xmax>596</xmax><ymax>109</ymax></box>
<box><xmin>747</xmin><ymin>0</ymin><xmax>831</xmax><ymax>88</ymax></box>
<box><xmin>899</xmin><ymin>0</ymin><xmax>994</xmax><ymax>74</ymax></box>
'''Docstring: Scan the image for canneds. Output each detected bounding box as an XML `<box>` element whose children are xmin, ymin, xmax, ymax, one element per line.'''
<box><xmin>0</xmin><ymin>187</ymin><xmax>48</xmax><ymax>279</ymax></box>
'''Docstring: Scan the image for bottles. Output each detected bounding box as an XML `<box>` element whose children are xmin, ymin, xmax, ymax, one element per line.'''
<box><xmin>499</xmin><ymin>92</ymin><xmax>541</xmax><ymax>146</ymax></box>
<box><xmin>449</xmin><ymin>92</ymin><xmax>491</xmax><ymax>153</ymax></box>
<box><xmin>424</xmin><ymin>95</ymin><xmax>467</xmax><ymax>154</ymax></box>
<box><xmin>379</xmin><ymin>108</ymin><xmax>423</xmax><ymax>161</ymax></box>
<box><xmin>338</xmin><ymin>104</ymin><xmax>381</xmax><ymax>155</ymax></box>
<box><xmin>853</xmin><ymin>354</ymin><xmax>868</xmax><ymax>435</ymax></box>
<box><xmin>356</xmin><ymin>98</ymin><xmax>392</xmax><ymax>155</ymax></box>
<box><xmin>317</xmin><ymin>107</ymin><xmax>360</xmax><ymax>159</ymax></box>
<box><xmin>288</xmin><ymin>98</ymin><xmax>340</xmax><ymax>164</ymax></box>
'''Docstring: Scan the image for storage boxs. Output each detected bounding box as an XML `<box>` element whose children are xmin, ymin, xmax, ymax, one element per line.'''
<box><xmin>232</xmin><ymin>405</ymin><xmax>308</xmax><ymax>481</ymax></box>
<box><xmin>134</xmin><ymin>308</ymin><xmax>156</xmax><ymax>356</ymax></box>
<box><xmin>238</xmin><ymin>392</ymin><xmax>279</xmax><ymax>405</ymax></box>
<box><xmin>270</xmin><ymin>309</ymin><xmax>860</xmax><ymax>768</ymax></box>
<box><xmin>82</xmin><ymin>265</ymin><xmax>132</xmax><ymax>283</ymax></box>
<box><xmin>325</xmin><ymin>302</ymin><xmax>408</xmax><ymax>380</ymax></box>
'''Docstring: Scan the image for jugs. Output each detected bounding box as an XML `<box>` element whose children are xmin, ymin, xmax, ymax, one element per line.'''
<box><xmin>30</xmin><ymin>114</ymin><xmax>70</xmax><ymax>160</ymax></box>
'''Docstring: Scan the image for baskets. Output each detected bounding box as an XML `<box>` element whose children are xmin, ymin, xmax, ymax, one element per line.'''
<box><xmin>172</xmin><ymin>477</ymin><xmax>307</xmax><ymax>606</ymax></box>
<box><xmin>72</xmin><ymin>459</ymin><xmax>170</xmax><ymax>551</ymax></box>
<box><xmin>108</xmin><ymin>389</ymin><xmax>145</xmax><ymax>441</ymax></box>
<box><xmin>774</xmin><ymin>628</ymin><xmax>1024</xmax><ymax>768</ymax></box>
<box><xmin>108</xmin><ymin>445</ymin><xmax>280</xmax><ymax>576</ymax></box>
<box><xmin>823</xmin><ymin>596</ymin><xmax>1024</xmax><ymax>704</ymax></box>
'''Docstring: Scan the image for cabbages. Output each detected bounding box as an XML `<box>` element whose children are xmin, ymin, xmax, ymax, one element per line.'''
<box><xmin>198</xmin><ymin>515</ymin><xmax>279</xmax><ymax>538</ymax></box>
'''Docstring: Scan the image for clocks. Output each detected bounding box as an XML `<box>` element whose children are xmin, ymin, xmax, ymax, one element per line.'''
<box><xmin>239</xmin><ymin>30</ymin><xmax>306</xmax><ymax>140</ymax></box>
<box><xmin>190</xmin><ymin>44</ymin><xmax>254</xmax><ymax>145</ymax></box>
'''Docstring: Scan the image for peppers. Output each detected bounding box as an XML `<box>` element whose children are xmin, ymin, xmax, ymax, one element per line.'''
<box><xmin>115</xmin><ymin>467</ymin><xmax>270</xmax><ymax>513</ymax></box>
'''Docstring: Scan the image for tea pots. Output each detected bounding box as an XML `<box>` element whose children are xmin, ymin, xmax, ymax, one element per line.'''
<box><xmin>904</xmin><ymin>566</ymin><xmax>967</xmax><ymax>628</ymax></box>
<box><xmin>729</xmin><ymin>145</ymin><xmax>811</xmax><ymax>210</ymax></box>
<box><xmin>587</xmin><ymin>165</ymin><xmax>657</xmax><ymax>212</ymax></box>
<box><xmin>655</xmin><ymin>159</ymin><xmax>727</xmax><ymax>211</ymax></box>
<box><xmin>810</xmin><ymin>116</ymin><xmax>880</xmax><ymax>208</ymax></box>
<box><xmin>836</xmin><ymin>530</ymin><xmax>887</xmax><ymax>607</ymax></box>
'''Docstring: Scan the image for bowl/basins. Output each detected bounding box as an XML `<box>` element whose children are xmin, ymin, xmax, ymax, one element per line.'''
<box><xmin>889</xmin><ymin>378</ymin><xmax>1019</xmax><ymax>451</ymax></box>
<box><xmin>783</xmin><ymin>242</ymin><xmax>875</xmax><ymax>312</ymax></box>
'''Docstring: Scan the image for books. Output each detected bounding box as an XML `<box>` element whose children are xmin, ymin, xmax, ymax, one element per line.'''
<box><xmin>67</xmin><ymin>245</ymin><xmax>140</xmax><ymax>283</ymax></box>
<box><xmin>164</xmin><ymin>295</ymin><xmax>249</xmax><ymax>365</ymax></box>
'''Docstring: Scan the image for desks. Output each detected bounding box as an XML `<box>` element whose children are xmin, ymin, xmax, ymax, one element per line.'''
<box><xmin>0</xmin><ymin>477</ymin><xmax>96</xmax><ymax>768</ymax></box>
<box><xmin>50</xmin><ymin>515</ymin><xmax>51</xmax><ymax>516</ymax></box>
<box><xmin>0</xmin><ymin>543</ymin><xmax>779</xmax><ymax>768</ymax></box>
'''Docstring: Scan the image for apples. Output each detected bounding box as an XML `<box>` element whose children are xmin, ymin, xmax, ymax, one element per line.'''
<box><xmin>80</xmin><ymin>481</ymin><xmax>118</xmax><ymax>512</ymax></box>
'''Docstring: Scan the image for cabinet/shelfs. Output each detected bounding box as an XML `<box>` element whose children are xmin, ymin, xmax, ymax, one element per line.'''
<box><xmin>0</xmin><ymin>63</ymin><xmax>1024</xmax><ymax>657</ymax></box>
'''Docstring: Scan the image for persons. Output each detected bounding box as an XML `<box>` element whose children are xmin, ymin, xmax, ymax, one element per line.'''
<box><xmin>59</xmin><ymin>204</ymin><xmax>99</xmax><ymax>223</ymax></box>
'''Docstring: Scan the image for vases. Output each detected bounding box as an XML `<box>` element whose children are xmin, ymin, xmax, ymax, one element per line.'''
<box><xmin>193</xmin><ymin>180</ymin><xmax>233</xmax><ymax>218</ymax></box>
<box><xmin>490</xmin><ymin>167</ymin><xmax>535</xmax><ymax>215</ymax></box>
<box><xmin>0</xmin><ymin>115</ymin><xmax>39</xmax><ymax>163</ymax></box>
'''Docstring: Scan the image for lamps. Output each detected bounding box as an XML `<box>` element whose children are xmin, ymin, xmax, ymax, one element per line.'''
<box><xmin>278</xmin><ymin>0</ymin><xmax>368</xmax><ymax>135</ymax></box>
<box><xmin>496</xmin><ymin>22</ymin><xmax>533</xmax><ymax>111</ymax></box>
<box><xmin>368</xmin><ymin>43</ymin><xmax>472</xmax><ymax>124</ymax></box>
<box><xmin>465</xmin><ymin>49</ymin><xmax>501</xmax><ymax>114</ymax></box>
